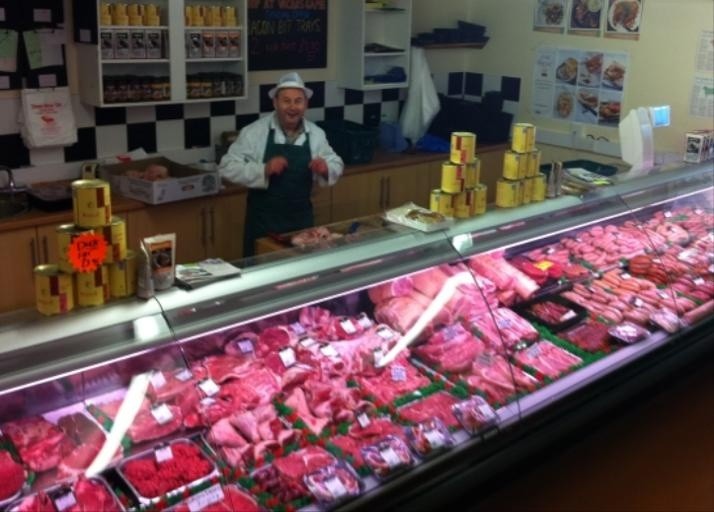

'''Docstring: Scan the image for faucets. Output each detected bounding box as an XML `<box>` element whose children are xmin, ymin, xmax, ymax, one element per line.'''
<box><xmin>0</xmin><ymin>164</ymin><xmax>27</xmax><ymax>193</ymax></box>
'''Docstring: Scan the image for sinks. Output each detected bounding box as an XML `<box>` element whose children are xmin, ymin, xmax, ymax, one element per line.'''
<box><xmin>1</xmin><ymin>196</ymin><xmax>30</xmax><ymax>219</ymax></box>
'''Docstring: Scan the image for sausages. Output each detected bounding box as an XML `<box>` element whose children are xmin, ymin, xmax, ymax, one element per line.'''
<box><xmin>527</xmin><ymin>209</ymin><xmax>714</xmax><ymax>334</ymax></box>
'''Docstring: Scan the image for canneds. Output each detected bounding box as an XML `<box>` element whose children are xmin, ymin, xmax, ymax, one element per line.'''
<box><xmin>32</xmin><ymin>178</ymin><xmax>137</xmax><ymax>316</ymax></box>
<box><xmin>495</xmin><ymin>122</ymin><xmax>545</xmax><ymax>208</ymax></box>
<box><xmin>430</xmin><ymin>131</ymin><xmax>487</xmax><ymax>218</ymax></box>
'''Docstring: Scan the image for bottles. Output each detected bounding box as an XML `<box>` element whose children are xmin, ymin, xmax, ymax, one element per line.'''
<box><xmin>103</xmin><ymin>73</ymin><xmax>243</xmax><ymax>101</ymax></box>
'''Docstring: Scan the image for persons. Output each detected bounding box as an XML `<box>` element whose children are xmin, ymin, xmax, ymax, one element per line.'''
<box><xmin>215</xmin><ymin>70</ymin><xmax>342</xmax><ymax>264</ymax></box>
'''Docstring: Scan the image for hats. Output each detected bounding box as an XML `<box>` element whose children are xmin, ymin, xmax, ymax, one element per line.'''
<box><xmin>267</xmin><ymin>72</ymin><xmax>314</xmax><ymax>101</ymax></box>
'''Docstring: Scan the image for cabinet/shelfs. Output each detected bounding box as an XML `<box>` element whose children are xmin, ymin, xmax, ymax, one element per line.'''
<box><xmin>336</xmin><ymin>0</ymin><xmax>413</xmax><ymax>92</ymax></box>
<box><xmin>78</xmin><ymin>0</ymin><xmax>249</xmax><ymax>109</ymax></box>
